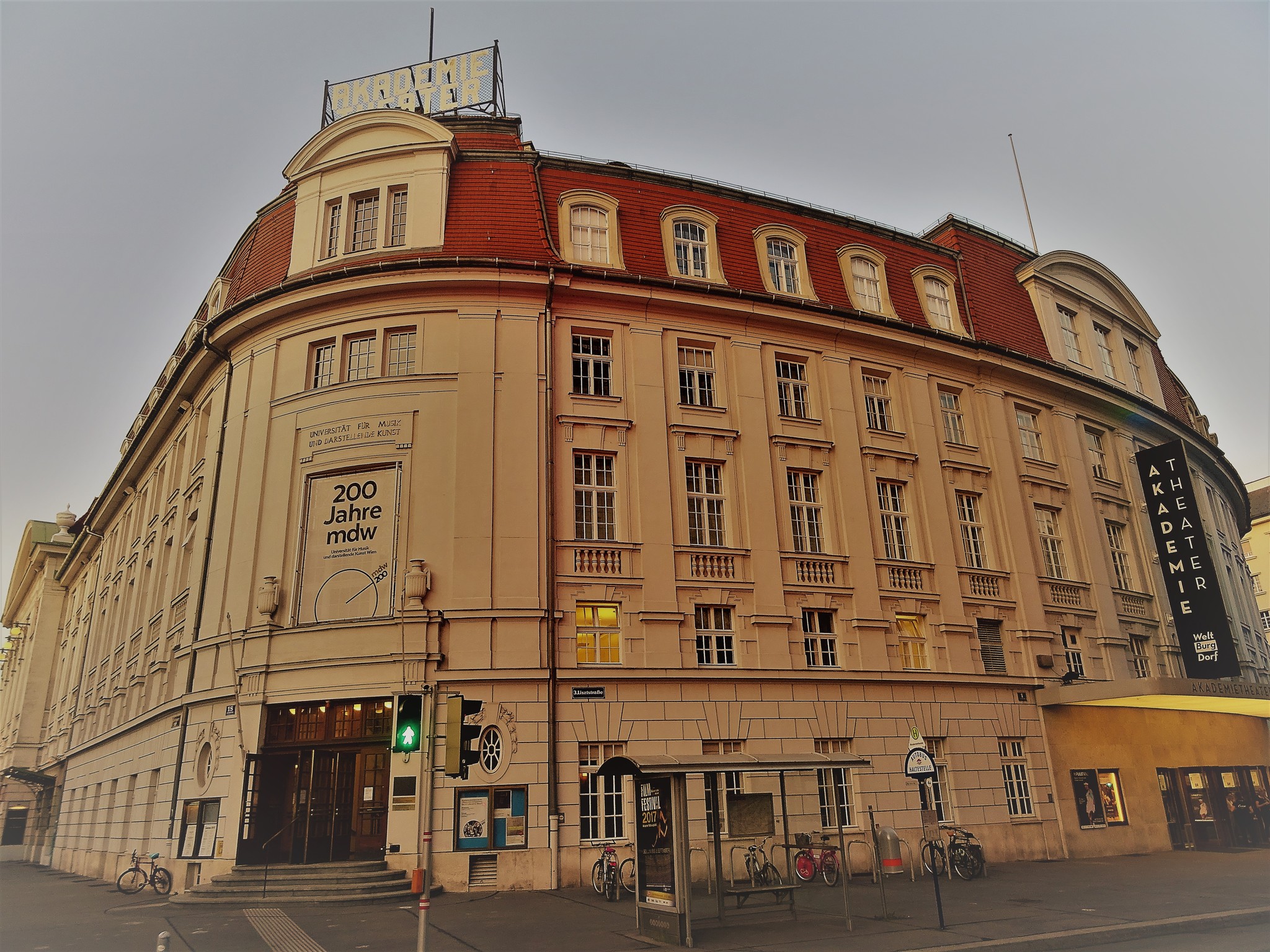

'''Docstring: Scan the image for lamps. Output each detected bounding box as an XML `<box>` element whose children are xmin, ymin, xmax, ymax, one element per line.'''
<box><xmin>3</xmin><ymin>635</ymin><xmax>22</xmax><ymax>649</ymax></box>
<box><xmin>8</xmin><ymin>621</ymin><xmax>30</xmax><ymax>635</ymax></box>
<box><xmin>0</xmin><ymin>648</ymin><xmax>17</xmax><ymax>660</ymax></box>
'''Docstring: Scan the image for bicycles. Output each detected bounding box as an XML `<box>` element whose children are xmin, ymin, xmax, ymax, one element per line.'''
<box><xmin>117</xmin><ymin>849</ymin><xmax>171</xmax><ymax>895</ymax></box>
<box><xmin>922</xmin><ymin>824</ymin><xmax>985</xmax><ymax>880</ymax></box>
<box><xmin>794</xmin><ymin>831</ymin><xmax>840</xmax><ymax>887</ymax></box>
<box><xmin>743</xmin><ymin>836</ymin><xmax>782</xmax><ymax>896</ymax></box>
<box><xmin>590</xmin><ymin>839</ymin><xmax>636</xmax><ymax>901</ymax></box>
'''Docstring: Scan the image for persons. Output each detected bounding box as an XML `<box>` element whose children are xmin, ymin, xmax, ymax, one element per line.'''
<box><xmin>1226</xmin><ymin>786</ymin><xmax>1270</xmax><ymax>846</ymax></box>
<box><xmin>1084</xmin><ymin>782</ymin><xmax>1095</xmax><ymax>825</ymax></box>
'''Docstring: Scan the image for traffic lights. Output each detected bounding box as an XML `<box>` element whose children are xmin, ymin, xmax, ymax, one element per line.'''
<box><xmin>396</xmin><ymin>695</ymin><xmax>422</xmax><ymax>752</ymax></box>
<box><xmin>445</xmin><ymin>694</ymin><xmax>482</xmax><ymax>780</ymax></box>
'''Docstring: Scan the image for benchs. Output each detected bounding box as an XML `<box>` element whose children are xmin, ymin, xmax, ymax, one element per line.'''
<box><xmin>726</xmin><ymin>884</ymin><xmax>802</xmax><ymax>909</ymax></box>
